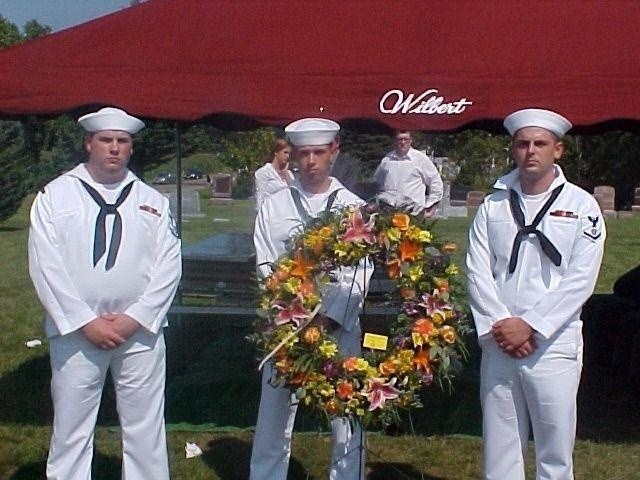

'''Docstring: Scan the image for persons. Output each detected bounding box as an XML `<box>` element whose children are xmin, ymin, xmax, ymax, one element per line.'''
<box><xmin>242</xmin><ymin>117</ymin><xmax>374</xmax><ymax>480</ymax></box>
<box><xmin>327</xmin><ymin>133</ymin><xmax>363</xmax><ymax>194</ymax></box>
<box><xmin>254</xmin><ymin>138</ymin><xmax>295</xmax><ymax>216</ymax></box>
<box><xmin>27</xmin><ymin>105</ymin><xmax>183</xmax><ymax>479</ymax></box>
<box><xmin>463</xmin><ymin>107</ymin><xmax>609</xmax><ymax>479</ymax></box>
<box><xmin>371</xmin><ymin>128</ymin><xmax>444</xmax><ymax>229</ymax></box>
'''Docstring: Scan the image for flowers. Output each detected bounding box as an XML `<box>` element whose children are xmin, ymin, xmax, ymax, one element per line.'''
<box><xmin>243</xmin><ymin>192</ymin><xmax>477</xmax><ymax>437</ymax></box>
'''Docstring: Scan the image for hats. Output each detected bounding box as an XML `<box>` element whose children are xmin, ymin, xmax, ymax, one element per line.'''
<box><xmin>78</xmin><ymin>108</ymin><xmax>145</xmax><ymax>134</ymax></box>
<box><xmin>503</xmin><ymin>109</ymin><xmax>573</xmax><ymax>138</ymax></box>
<box><xmin>285</xmin><ymin>118</ymin><xmax>341</xmax><ymax>146</ymax></box>
